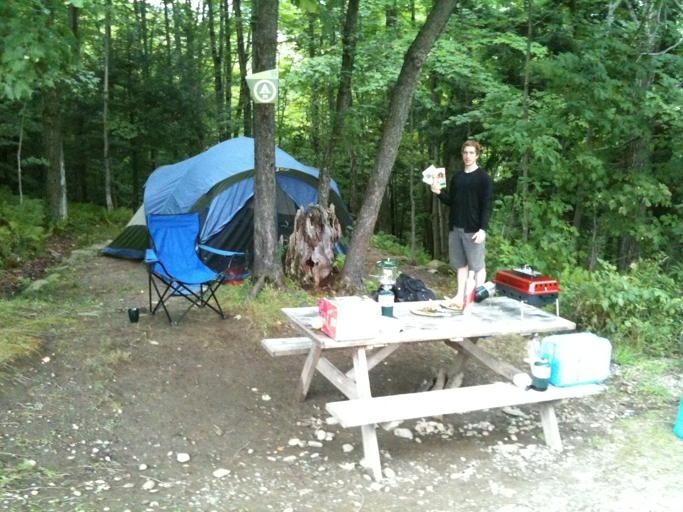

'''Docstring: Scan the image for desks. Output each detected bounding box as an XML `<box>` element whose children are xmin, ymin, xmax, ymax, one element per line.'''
<box><xmin>281</xmin><ymin>295</ymin><xmax>577</xmax><ymax>482</ymax></box>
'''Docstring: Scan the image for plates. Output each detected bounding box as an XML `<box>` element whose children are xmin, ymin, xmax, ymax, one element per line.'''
<box><xmin>409</xmin><ymin>304</ymin><xmax>461</xmax><ymax>316</ymax></box>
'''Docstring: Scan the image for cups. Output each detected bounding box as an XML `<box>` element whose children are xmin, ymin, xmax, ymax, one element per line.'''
<box><xmin>377</xmin><ymin>289</ymin><xmax>394</xmax><ymax>317</ymax></box>
<box><xmin>473</xmin><ymin>280</ymin><xmax>496</xmax><ymax>302</ymax></box>
<box><xmin>530</xmin><ymin>364</ymin><xmax>552</xmax><ymax>391</ymax></box>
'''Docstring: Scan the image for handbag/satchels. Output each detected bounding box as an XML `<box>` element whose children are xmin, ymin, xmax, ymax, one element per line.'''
<box><xmin>394</xmin><ymin>274</ymin><xmax>436</xmax><ymax>301</ymax></box>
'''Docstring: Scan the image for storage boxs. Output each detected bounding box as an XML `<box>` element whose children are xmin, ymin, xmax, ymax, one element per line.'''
<box><xmin>540</xmin><ymin>332</ymin><xmax>612</xmax><ymax>386</ymax></box>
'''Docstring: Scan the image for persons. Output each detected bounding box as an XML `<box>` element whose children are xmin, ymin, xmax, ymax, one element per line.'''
<box><xmin>430</xmin><ymin>139</ymin><xmax>494</xmax><ymax>301</ymax></box>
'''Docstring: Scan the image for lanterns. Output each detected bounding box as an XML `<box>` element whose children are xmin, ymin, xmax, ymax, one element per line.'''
<box><xmin>102</xmin><ymin>138</ymin><xmax>350</xmax><ymax>296</ymax></box>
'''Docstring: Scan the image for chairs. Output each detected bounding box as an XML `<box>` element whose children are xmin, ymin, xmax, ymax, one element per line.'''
<box><xmin>142</xmin><ymin>212</ymin><xmax>245</xmax><ymax>327</ymax></box>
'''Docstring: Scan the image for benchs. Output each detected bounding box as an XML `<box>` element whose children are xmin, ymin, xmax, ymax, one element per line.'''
<box><xmin>259</xmin><ymin>337</ymin><xmax>321</xmax><ymax>404</ymax></box>
<box><xmin>325</xmin><ymin>379</ymin><xmax>604</xmax><ymax>483</ymax></box>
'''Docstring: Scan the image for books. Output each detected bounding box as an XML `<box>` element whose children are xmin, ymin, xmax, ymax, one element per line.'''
<box><xmin>422</xmin><ymin>164</ymin><xmax>437</xmax><ymax>184</ymax></box>
<box><xmin>431</xmin><ymin>167</ymin><xmax>447</xmax><ymax>190</ymax></box>
<box><xmin>422</xmin><ymin>177</ymin><xmax>430</xmax><ymax>186</ymax></box>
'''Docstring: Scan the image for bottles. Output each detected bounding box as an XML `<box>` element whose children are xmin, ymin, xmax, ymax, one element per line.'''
<box><xmin>463</xmin><ymin>270</ymin><xmax>476</xmax><ymax>308</ymax></box>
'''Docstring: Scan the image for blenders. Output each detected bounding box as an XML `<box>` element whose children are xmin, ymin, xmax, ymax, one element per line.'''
<box><xmin>372</xmin><ymin>256</ymin><xmax>398</xmax><ymax>303</ymax></box>
